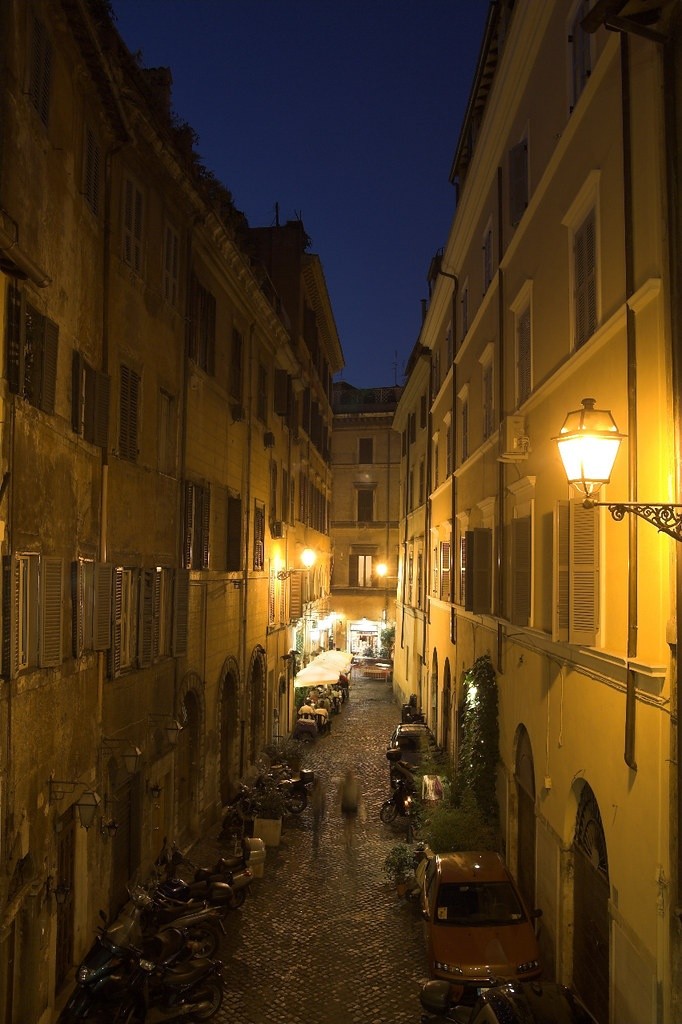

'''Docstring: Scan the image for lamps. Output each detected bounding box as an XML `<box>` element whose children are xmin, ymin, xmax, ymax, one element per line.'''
<box><xmin>45</xmin><ymin>875</ymin><xmax>73</xmax><ymax>906</ymax></box>
<box><xmin>146</xmin><ymin>779</ymin><xmax>163</xmax><ymax>799</ymax></box>
<box><xmin>101</xmin><ymin>815</ymin><xmax>119</xmax><ymax>838</ymax></box>
<box><xmin>550</xmin><ymin>399</ymin><xmax>681</xmax><ymax>544</ymax></box>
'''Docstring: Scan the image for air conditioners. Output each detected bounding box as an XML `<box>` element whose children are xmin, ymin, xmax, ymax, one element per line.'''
<box><xmin>497</xmin><ymin>415</ymin><xmax>528</xmax><ymax>463</ymax></box>
<box><xmin>265</xmin><ymin>431</ymin><xmax>275</xmax><ymax>448</ymax></box>
<box><xmin>274</xmin><ymin>522</ymin><xmax>287</xmax><ymax>540</ymax></box>
<box><xmin>233</xmin><ymin>403</ymin><xmax>247</xmax><ymax>423</ymax></box>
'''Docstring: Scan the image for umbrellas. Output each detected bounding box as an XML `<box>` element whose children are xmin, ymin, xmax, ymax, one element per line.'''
<box><xmin>294</xmin><ymin>650</ymin><xmax>354</xmax><ymax>687</ymax></box>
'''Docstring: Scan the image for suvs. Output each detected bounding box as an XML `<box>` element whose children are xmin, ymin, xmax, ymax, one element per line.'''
<box><xmin>471</xmin><ymin>982</ymin><xmax>598</xmax><ymax>1023</ymax></box>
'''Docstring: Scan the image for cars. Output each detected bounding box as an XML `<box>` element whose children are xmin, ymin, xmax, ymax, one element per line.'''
<box><xmin>388</xmin><ymin>723</ymin><xmax>439</xmax><ymax>771</ymax></box>
<box><xmin>421</xmin><ymin>851</ymin><xmax>546</xmax><ymax>984</ymax></box>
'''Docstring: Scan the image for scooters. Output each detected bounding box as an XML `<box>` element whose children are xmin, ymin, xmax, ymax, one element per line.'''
<box><xmin>51</xmin><ymin>760</ymin><xmax>314</xmax><ymax>1024</ymax></box>
<box><xmin>380</xmin><ymin>749</ymin><xmax>429</xmax><ymax>834</ymax></box>
<box><xmin>420</xmin><ymin>980</ymin><xmax>472</xmax><ymax>1024</ymax></box>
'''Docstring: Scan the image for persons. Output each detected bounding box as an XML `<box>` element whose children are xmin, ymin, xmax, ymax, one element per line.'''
<box><xmin>298</xmin><ymin>675</ymin><xmax>349</xmax><ymax>732</ymax></box>
